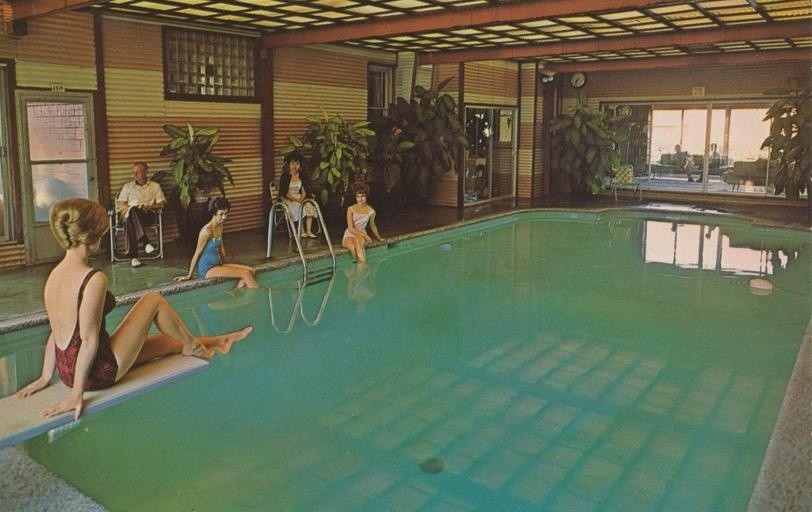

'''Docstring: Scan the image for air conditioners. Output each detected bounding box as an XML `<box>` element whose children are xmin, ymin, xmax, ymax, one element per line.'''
<box><xmin>149</xmin><ymin>122</ymin><xmax>235</xmax><ymax>254</ymax></box>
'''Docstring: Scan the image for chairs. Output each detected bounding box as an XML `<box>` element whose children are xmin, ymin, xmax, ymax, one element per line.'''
<box><xmin>270</xmin><ymin>179</ymin><xmax>322</xmax><ymax>239</ymax></box>
<box><xmin>108</xmin><ymin>208</ymin><xmax>163</xmax><ymax>262</ymax></box>
<box><xmin>612</xmin><ymin>165</ymin><xmax>643</xmax><ymax>202</ymax></box>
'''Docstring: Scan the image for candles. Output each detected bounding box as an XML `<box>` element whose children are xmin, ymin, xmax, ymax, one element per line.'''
<box><xmin>549</xmin><ymin>96</ymin><xmax>621</xmax><ymax>196</ymax></box>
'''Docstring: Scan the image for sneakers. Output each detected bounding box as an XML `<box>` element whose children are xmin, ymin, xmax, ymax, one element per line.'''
<box><xmin>301</xmin><ymin>232</ymin><xmax>319</xmax><ymax>239</ymax></box>
<box><xmin>131</xmin><ymin>258</ymin><xmax>142</xmax><ymax>267</ymax></box>
<box><xmin>144</xmin><ymin>243</ymin><xmax>155</xmax><ymax>254</ymax></box>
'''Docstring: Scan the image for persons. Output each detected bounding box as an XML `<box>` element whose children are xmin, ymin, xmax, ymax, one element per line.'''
<box><xmin>115</xmin><ymin>160</ymin><xmax>168</xmax><ymax>268</ymax></box>
<box><xmin>15</xmin><ymin>194</ymin><xmax>255</xmax><ymax>419</ymax></box>
<box><xmin>278</xmin><ymin>150</ymin><xmax>320</xmax><ymax>238</ymax></box>
<box><xmin>187</xmin><ymin>288</ymin><xmax>259</xmax><ymax>340</ymax></box>
<box><xmin>339</xmin><ymin>182</ymin><xmax>386</xmax><ymax>263</ymax></box>
<box><xmin>341</xmin><ymin>261</ymin><xmax>381</xmax><ymax>324</ymax></box>
<box><xmin>173</xmin><ymin>196</ymin><xmax>285</xmax><ymax>298</ymax></box>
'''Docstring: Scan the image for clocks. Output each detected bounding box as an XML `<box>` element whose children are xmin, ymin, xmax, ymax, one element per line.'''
<box><xmin>569</xmin><ymin>72</ymin><xmax>587</xmax><ymax>89</ymax></box>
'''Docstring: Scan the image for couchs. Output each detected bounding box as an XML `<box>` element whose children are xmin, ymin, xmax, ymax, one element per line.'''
<box><xmin>651</xmin><ymin>154</ymin><xmax>728</xmax><ymax>179</ymax></box>
